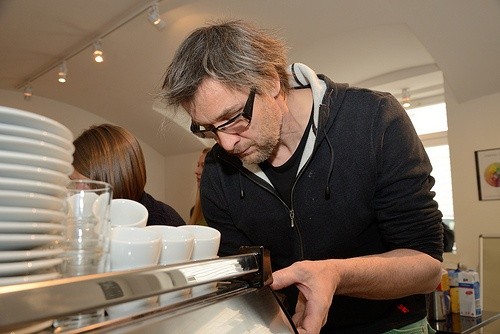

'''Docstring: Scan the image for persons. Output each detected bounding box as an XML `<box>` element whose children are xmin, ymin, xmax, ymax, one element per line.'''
<box><xmin>160</xmin><ymin>18</ymin><xmax>445</xmax><ymax>334</ymax></box>
<box><xmin>187</xmin><ymin>146</ymin><xmax>213</xmax><ymax>227</ymax></box>
<box><xmin>69</xmin><ymin>123</ymin><xmax>186</xmax><ymax>230</ymax></box>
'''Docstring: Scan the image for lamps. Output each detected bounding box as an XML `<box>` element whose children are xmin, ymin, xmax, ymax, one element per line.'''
<box><xmin>23</xmin><ymin>80</ymin><xmax>33</xmax><ymax>96</ymax></box>
<box><xmin>57</xmin><ymin>60</ymin><xmax>67</xmax><ymax>84</ymax></box>
<box><xmin>92</xmin><ymin>39</ymin><xmax>104</xmax><ymax>62</ymax></box>
<box><xmin>402</xmin><ymin>88</ymin><xmax>410</xmax><ymax>107</ymax></box>
<box><xmin>147</xmin><ymin>3</ymin><xmax>166</xmax><ymax>31</ymax></box>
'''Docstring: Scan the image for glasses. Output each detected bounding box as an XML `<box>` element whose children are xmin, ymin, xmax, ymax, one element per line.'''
<box><xmin>190</xmin><ymin>89</ymin><xmax>256</xmax><ymax>138</ymax></box>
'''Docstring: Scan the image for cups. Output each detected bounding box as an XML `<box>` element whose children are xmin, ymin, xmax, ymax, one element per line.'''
<box><xmin>425</xmin><ymin>291</ymin><xmax>451</xmax><ymax>321</ymax></box>
<box><xmin>49</xmin><ymin>177</ymin><xmax>222</xmax><ymax>327</ymax></box>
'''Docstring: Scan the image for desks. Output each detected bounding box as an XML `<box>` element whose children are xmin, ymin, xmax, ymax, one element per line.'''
<box><xmin>428</xmin><ymin>311</ymin><xmax>500</xmax><ymax>334</ymax></box>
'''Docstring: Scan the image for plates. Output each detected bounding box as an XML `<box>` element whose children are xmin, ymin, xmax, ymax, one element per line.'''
<box><xmin>0</xmin><ymin>105</ymin><xmax>76</xmax><ymax>334</ymax></box>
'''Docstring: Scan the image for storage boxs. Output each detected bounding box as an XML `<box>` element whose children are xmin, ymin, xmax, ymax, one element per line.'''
<box><xmin>447</xmin><ymin>269</ymin><xmax>482</xmax><ymax>317</ymax></box>
